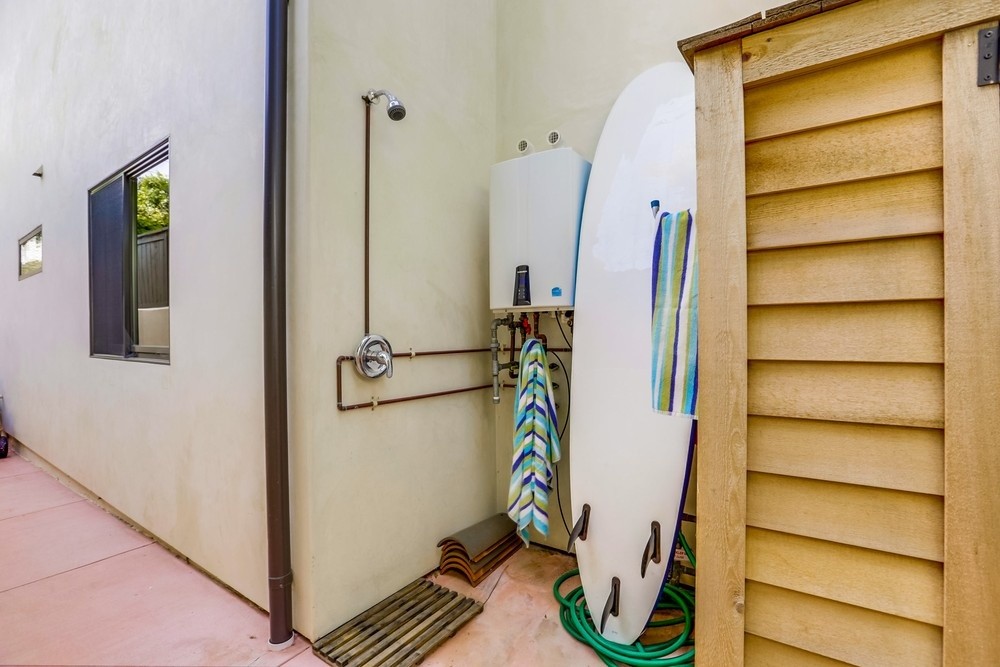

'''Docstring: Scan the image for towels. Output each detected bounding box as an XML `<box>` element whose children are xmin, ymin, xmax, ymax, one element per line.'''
<box><xmin>505</xmin><ymin>338</ymin><xmax>561</xmax><ymax>549</ymax></box>
<box><xmin>650</xmin><ymin>207</ymin><xmax>698</xmax><ymax>421</ymax></box>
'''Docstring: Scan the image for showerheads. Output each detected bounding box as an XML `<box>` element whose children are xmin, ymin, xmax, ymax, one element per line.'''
<box><xmin>362</xmin><ymin>87</ymin><xmax>407</xmax><ymax>122</ymax></box>
